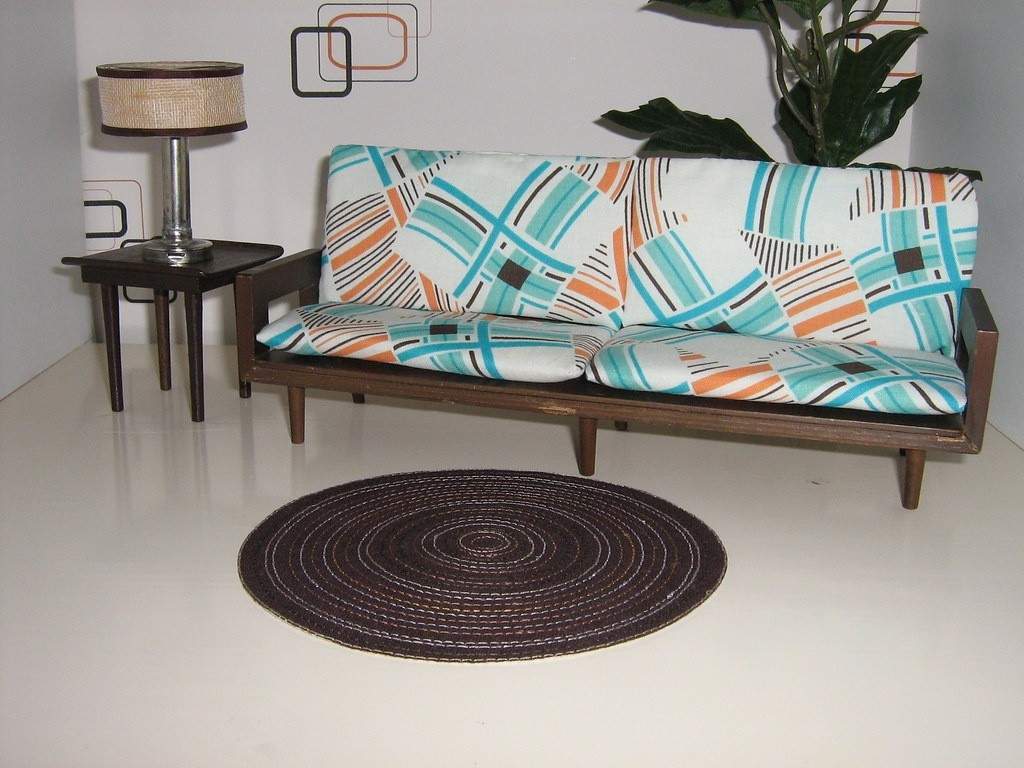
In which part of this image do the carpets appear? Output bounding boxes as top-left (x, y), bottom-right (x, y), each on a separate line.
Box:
top-left (237, 467), bottom-right (729, 662)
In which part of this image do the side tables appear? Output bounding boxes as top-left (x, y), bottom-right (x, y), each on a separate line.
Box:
top-left (61, 239), bottom-right (284, 422)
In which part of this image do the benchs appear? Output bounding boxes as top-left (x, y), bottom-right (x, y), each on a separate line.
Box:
top-left (235, 145), bottom-right (999, 510)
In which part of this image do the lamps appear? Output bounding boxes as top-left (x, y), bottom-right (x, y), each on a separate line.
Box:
top-left (96, 58), bottom-right (248, 263)
